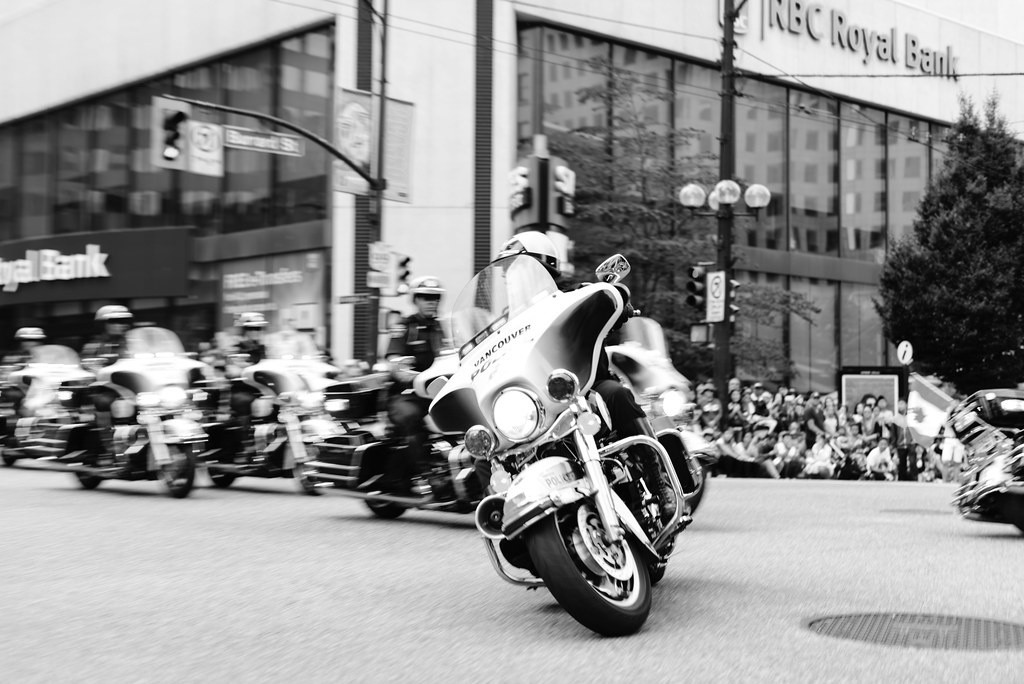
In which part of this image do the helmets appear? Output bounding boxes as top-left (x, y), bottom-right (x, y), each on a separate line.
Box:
top-left (14, 328), bottom-right (45, 339)
top-left (408, 279), bottom-right (443, 296)
top-left (94, 305), bottom-right (133, 321)
top-left (235, 313), bottom-right (265, 328)
top-left (496, 232), bottom-right (563, 275)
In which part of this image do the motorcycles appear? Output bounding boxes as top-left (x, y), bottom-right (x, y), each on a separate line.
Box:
top-left (425, 250), bottom-right (704, 637)
top-left (947, 389), bottom-right (1024, 529)
top-left (0, 327), bottom-right (478, 519)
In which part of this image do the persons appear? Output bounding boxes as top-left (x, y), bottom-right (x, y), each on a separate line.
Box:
top-left (694, 376), bottom-right (928, 482)
top-left (0, 326), bottom-right (58, 435)
top-left (932, 424), bottom-right (950, 468)
top-left (494, 230), bottom-right (680, 532)
top-left (198, 309), bottom-right (330, 466)
top-left (79, 304), bottom-right (182, 453)
top-left (385, 276), bottom-right (456, 497)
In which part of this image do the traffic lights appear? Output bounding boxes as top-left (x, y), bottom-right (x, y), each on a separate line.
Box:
top-left (686, 264), bottom-right (710, 308)
top-left (725, 272), bottom-right (741, 320)
top-left (393, 252), bottom-right (411, 293)
top-left (152, 97), bottom-right (193, 171)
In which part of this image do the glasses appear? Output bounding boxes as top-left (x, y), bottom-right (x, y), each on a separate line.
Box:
top-left (417, 294), bottom-right (440, 301)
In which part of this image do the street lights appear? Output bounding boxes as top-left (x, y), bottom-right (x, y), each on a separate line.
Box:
top-left (678, 0), bottom-right (774, 409)
